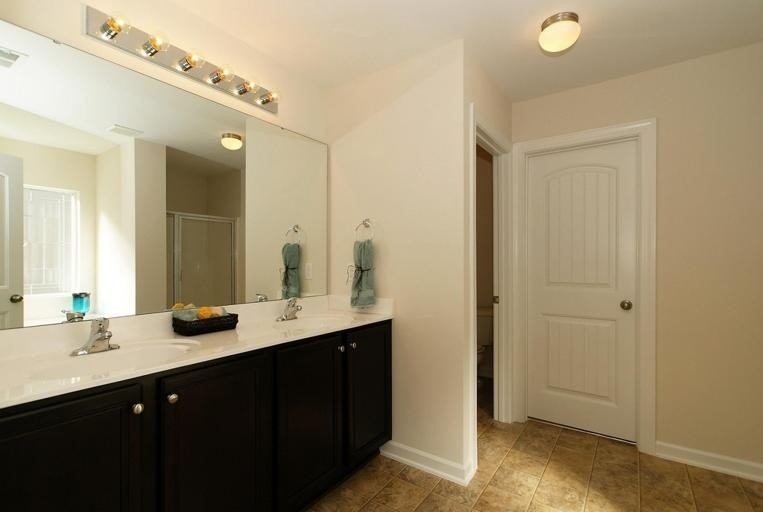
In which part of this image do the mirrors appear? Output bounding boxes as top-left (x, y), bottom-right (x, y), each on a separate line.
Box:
top-left (0, 18), bottom-right (334, 329)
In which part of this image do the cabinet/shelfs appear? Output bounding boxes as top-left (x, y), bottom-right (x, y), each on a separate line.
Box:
top-left (0, 347), bottom-right (279, 511)
top-left (277, 317), bottom-right (392, 512)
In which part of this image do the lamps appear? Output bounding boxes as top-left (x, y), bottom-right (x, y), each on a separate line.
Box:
top-left (222, 132), bottom-right (245, 150)
top-left (88, 6), bottom-right (282, 113)
top-left (536, 11), bottom-right (580, 55)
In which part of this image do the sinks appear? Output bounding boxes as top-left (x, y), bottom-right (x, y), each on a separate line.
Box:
top-left (254, 314), bottom-right (353, 342)
top-left (0, 338), bottom-right (199, 403)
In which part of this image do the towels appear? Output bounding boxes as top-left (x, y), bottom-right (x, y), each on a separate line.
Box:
top-left (277, 242), bottom-right (302, 299)
top-left (350, 242), bottom-right (375, 306)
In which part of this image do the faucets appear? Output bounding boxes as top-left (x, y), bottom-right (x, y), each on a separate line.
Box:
top-left (276, 296), bottom-right (306, 321)
top-left (72, 316), bottom-right (122, 356)
top-left (255, 293), bottom-right (268, 303)
top-left (58, 308), bottom-right (86, 324)
